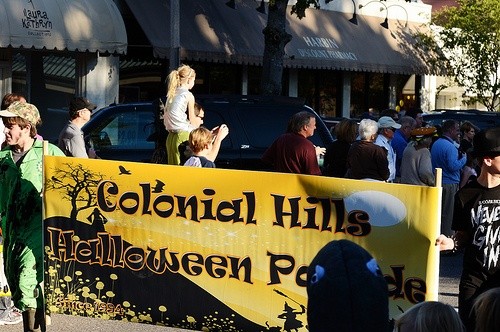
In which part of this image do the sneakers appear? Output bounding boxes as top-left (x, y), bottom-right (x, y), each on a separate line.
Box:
top-left (1, 308), bottom-right (23, 325)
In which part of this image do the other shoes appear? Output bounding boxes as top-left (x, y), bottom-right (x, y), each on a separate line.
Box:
top-left (440, 250), bottom-right (458, 256)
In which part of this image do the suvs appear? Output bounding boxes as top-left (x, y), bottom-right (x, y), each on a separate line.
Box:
top-left (421, 108), bottom-right (500, 145)
top-left (80, 96), bottom-right (334, 174)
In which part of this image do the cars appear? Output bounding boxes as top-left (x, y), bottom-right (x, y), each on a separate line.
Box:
top-left (323, 116), bottom-right (361, 140)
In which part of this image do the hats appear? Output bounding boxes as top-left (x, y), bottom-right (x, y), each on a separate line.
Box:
top-left (473, 127), bottom-right (500, 154)
top-left (0, 101), bottom-right (40, 125)
top-left (378, 116), bottom-right (402, 129)
top-left (410, 126), bottom-right (439, 141)
top-left (69, 96), bottom-right (97, 111)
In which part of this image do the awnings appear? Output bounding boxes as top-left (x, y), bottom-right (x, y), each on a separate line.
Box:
top-left (0, 0), bottom-right (127, 56)
top-left (118, 0), bottom-right (455, 77)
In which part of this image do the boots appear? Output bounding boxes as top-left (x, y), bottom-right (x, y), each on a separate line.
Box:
top-left (22, 310), bottom-right (42, 332)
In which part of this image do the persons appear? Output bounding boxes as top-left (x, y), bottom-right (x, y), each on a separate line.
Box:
top-left (436, 129), bottom-right (500, 324)
top-left (0, 101), bottom-right (66, 332)
top-left (0, 94), bottom-right (26, 324)
top-left (468, 288), bottom-right (500, 332)
top-left (164, 66), bottom-right (197, 133)
top-left (60, 97), bottom-right (97, 159)
top-left (178, 127), bottom-right (215, 167)
top-left (166, 104), bottom-right (228, 164)
top-left (267, 112), bottom-right (322, 175)
top-left (322, 105), bottom-right (475, 257)
top-left (395, 302), bottom-right (464, 332)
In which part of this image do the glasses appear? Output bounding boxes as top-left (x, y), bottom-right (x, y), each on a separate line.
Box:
top-left (471, 131), bottom-right (476, 133)
top-left (387, 127), bottom-right (396, 132)
top-left (197, 115), bottom-right (204, 120)
